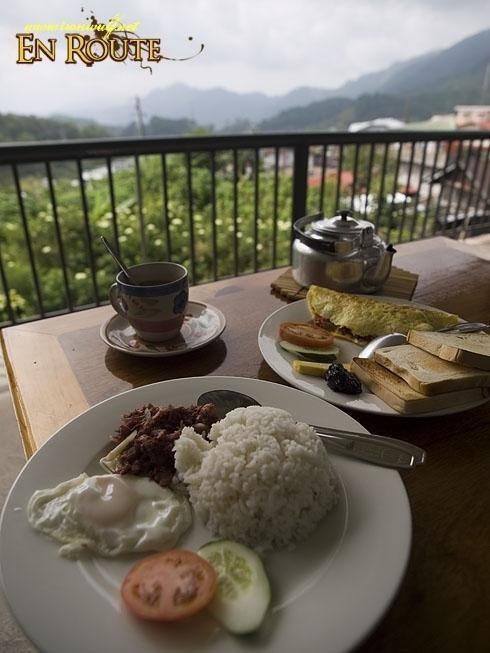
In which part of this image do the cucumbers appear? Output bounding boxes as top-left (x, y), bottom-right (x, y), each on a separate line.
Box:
top-left (190, 539), bottom-right (273, 636)
top-left (280, 340), bottom-right (340, 360)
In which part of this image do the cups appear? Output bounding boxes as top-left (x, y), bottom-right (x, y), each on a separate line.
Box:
top-left (107, 262), bottom-right (190, 340)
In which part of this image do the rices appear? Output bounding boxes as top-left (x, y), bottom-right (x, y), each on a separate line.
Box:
top-left (171, 404), bottom-right (340, 557)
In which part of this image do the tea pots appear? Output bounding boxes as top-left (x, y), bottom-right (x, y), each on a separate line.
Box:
top-left (289, 208), bottom-right (398, 294)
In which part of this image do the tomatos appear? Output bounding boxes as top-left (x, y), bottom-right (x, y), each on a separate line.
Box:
top-left (122, 548), bottom-right (218, 621)
top-left (279, 321), bottom-right (333, 348)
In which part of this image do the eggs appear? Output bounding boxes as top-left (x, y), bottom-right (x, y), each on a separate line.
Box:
top-left (29, 470), bottom-right (193, 560)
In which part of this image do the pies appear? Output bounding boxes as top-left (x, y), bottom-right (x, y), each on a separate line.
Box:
top-left (307, 284), bottom-right (459, 348)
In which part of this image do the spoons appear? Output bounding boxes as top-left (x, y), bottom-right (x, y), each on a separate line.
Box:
top-left (197, 387), bottom-right (428, 470)
top-left (356, 321), bottom-right (487, 361)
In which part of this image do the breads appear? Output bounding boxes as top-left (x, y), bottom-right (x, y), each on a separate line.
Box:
top-left (352, 329), bottom-right (490, 415)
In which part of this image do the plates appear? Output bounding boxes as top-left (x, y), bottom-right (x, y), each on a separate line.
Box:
top-left (2, 374), bottom-right (416, 650)
top-left (253, 292), bottom-right (490, 416)
top-left (97, 299), bottom-right (227, 360)
